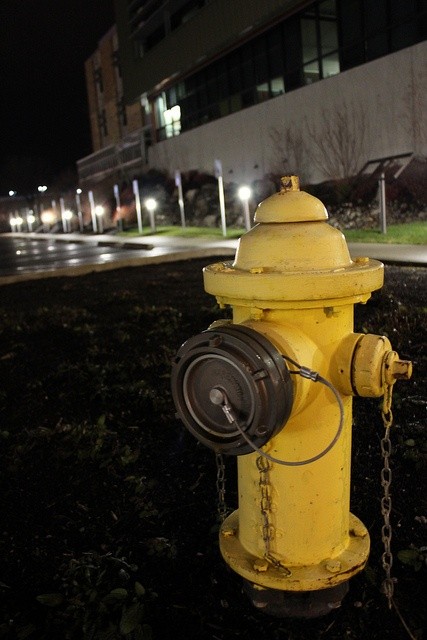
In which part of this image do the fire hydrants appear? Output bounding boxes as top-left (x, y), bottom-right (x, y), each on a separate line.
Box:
top-left (169, 173), bottom-right (414, 622)
